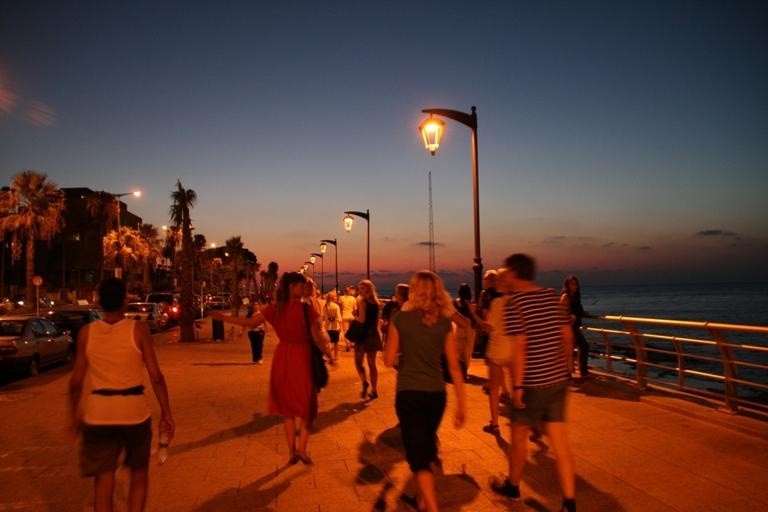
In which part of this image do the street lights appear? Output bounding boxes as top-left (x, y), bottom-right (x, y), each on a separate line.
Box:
top-left (111, 193), bottom-right (138, 250)
top-left (298, 239), bottom-right (338, 298)
top-left (419, 106), bottom-right (483, 302)
top-left (343, 210), bottom-right (370, 280)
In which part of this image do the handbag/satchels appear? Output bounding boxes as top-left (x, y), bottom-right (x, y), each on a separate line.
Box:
top-left (306, 335), bottom-right (329, 388)
top-left (345, 320), bottom-right (364, 345)
top-left (328, 313), bottom-right (335, 321)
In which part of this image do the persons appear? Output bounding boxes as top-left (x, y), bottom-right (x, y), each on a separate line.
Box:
top-left (207, 251), bottom-right (588, 510)
top-left (67, 277), bottom-right (175, 511)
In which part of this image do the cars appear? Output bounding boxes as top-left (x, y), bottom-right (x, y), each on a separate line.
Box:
top-left (122, 291), bottom-right (233, 331)
top-left (0, 307), bottom-right (104, 377)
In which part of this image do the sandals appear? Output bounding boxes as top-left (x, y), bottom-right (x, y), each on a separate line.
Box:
top-left (483, 423), bottom-right (500, 435)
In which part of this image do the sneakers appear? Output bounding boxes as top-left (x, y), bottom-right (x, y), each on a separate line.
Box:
top-left (288, 450), bottom-right (312, 464)
top-left (492, 480), bottom-right (520, 501)
top-left (361, 380), bottom-right (378, 399)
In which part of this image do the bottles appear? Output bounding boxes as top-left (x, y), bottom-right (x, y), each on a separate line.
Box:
top-left (158, 432), bottom-right (169, 466)
